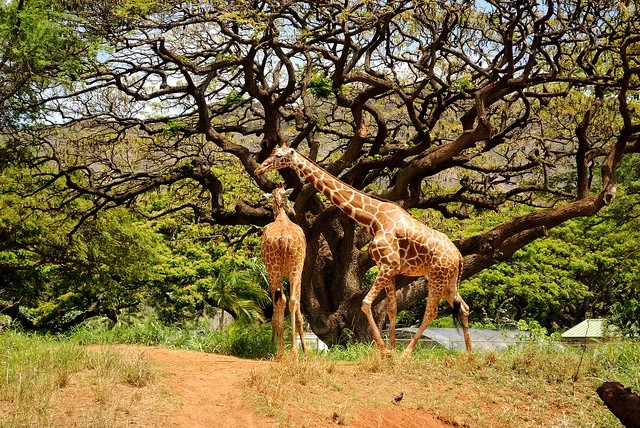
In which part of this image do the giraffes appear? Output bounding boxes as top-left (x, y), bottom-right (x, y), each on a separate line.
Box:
top-left (261, 182), bottom-right (307, 360)
top-left (253, 140), bottom-right (472, 361)
